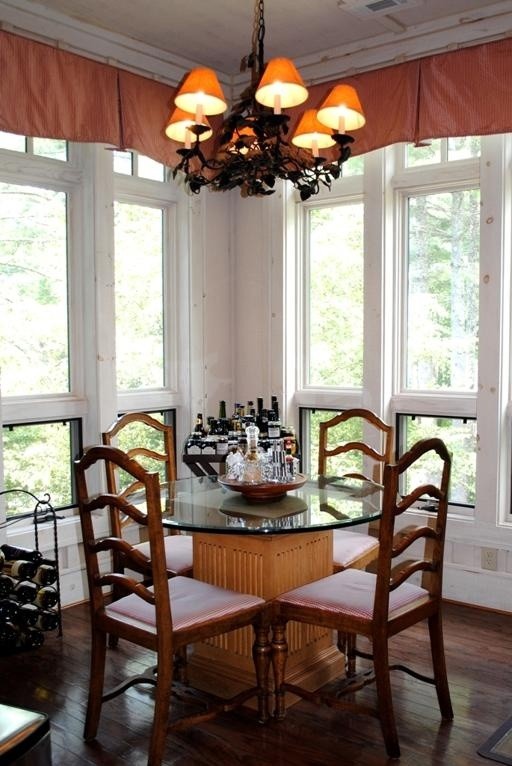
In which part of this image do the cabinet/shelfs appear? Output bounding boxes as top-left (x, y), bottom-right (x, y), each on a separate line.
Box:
top-left (0, 489), bottom-right (63, 652)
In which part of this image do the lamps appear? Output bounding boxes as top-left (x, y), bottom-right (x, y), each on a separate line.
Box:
top-left (164, 0), bottom-right (366, 202)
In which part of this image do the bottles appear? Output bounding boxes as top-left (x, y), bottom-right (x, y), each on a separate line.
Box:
top-left (185, 395), bottom-right (297, 457)
top-left (226, 423), bottom-right (295, 484)
top-left (0, 544), bottom-right (59, 655)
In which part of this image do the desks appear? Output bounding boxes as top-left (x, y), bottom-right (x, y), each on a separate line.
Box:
top-left (183, 454), bottom-right (300, 476)
top-left (124, 475), bottom-right (402, 718)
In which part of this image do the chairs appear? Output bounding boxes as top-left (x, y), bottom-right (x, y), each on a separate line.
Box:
top-left (102, 413), bottom-right (194, 662)
top-left (75, 446), bottom-right (268, 766)
top-left (318, 409), bottom-right (394, 654)
top-left (269, 438), bottom-right (454, 758)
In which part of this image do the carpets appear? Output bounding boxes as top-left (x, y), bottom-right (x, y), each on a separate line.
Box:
top-left (477, 716), bottom-right (512, 766)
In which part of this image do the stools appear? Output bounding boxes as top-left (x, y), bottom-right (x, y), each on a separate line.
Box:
top-left (0, 702), bottom-right (52, 766)
